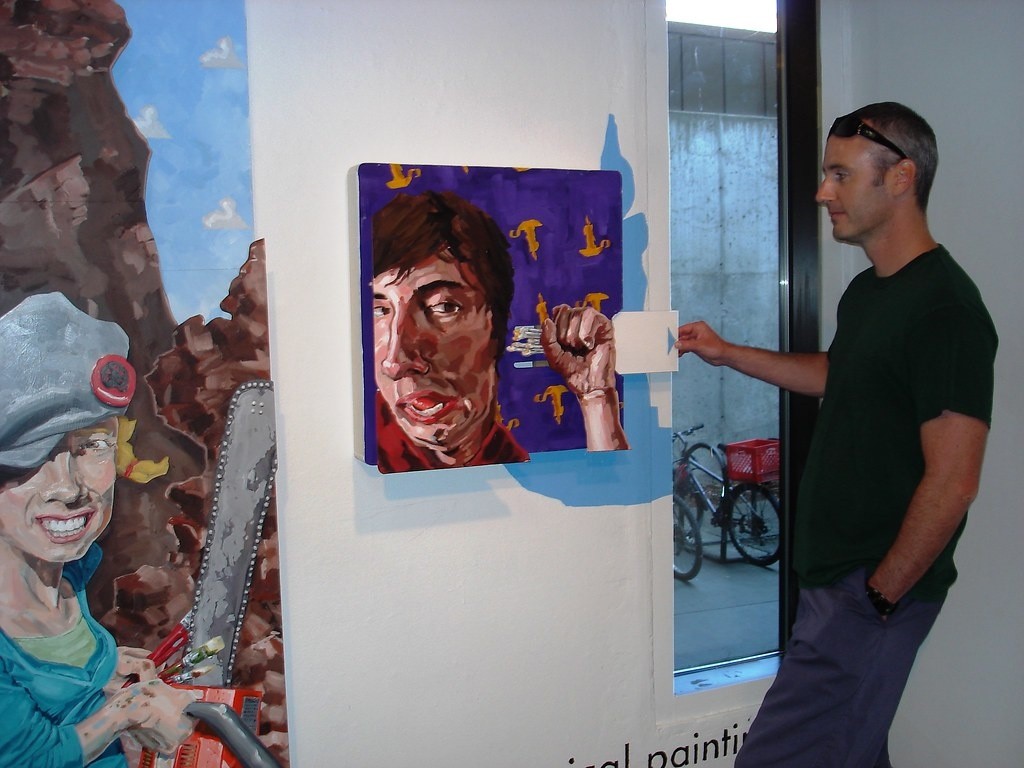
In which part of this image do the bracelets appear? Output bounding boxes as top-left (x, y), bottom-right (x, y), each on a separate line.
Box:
top-left (866, 584), bottom-right (897, 616)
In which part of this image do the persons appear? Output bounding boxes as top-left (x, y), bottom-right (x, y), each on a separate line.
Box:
top-left (675, 100), bottom-right (1000, 768)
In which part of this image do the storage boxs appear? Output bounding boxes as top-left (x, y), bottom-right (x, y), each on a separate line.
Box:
top-left (726, 439), bottom-right (779, 484)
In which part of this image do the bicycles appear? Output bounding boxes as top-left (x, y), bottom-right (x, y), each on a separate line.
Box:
top-left (672, 424), bottom-right (783, 581)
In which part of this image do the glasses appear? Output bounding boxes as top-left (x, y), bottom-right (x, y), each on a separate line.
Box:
top-left (827, 117), bottom-right (907, 160)
